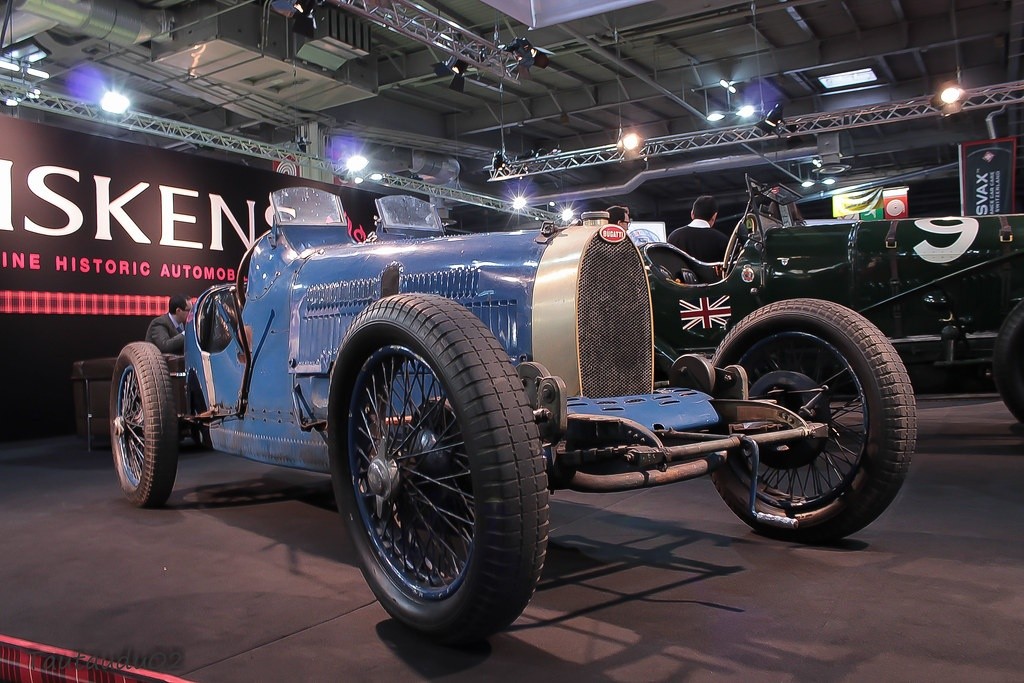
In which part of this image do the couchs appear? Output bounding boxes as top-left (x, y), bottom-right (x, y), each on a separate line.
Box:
top-left (71, 353), bottom-right (186, 453)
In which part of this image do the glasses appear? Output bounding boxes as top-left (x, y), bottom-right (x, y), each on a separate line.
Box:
top-left (622, 221), bottom-right (631, 227)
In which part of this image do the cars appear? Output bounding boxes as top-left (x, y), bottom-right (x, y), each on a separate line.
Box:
top-left (632, 211), bottom-right (1024, 429)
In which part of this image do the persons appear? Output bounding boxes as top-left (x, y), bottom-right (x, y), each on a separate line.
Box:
top-left (606, 205), bottom-right (630, 233)
top-left (667, 196), bottom-right (730, 264)
top-left (145, 295), bottom-right (193, 356)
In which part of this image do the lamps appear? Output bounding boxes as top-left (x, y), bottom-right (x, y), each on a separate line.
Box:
top-left (293, 0), bottom-right (316, 16)
top-left (765, 103), bottom-right (786, 127)
top-left (450, 59), bottom-right (469, 75)
top-left (801, 177), bottom-right (836, 188)
top-left (503, 37), bottom-right (555, 69)
